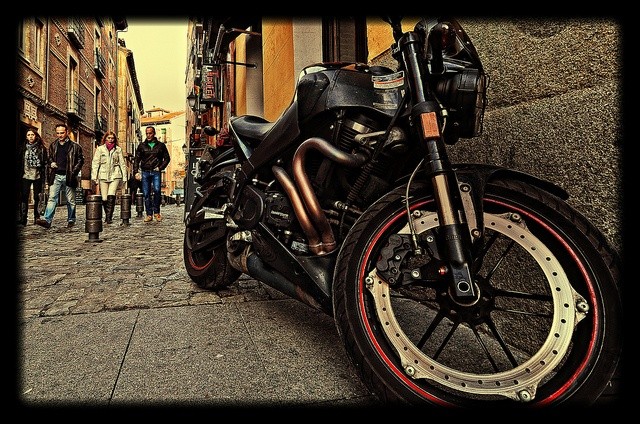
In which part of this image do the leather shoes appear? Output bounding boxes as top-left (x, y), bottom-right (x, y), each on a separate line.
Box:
top-left (36, 218), bottom-right (51, 228)
top-left (68, 220), bottom-right (75, 227)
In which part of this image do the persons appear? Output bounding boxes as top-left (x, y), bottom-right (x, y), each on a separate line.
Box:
top-left (133, 127), bottom-right (170, 222)
top-left (16, 128), bottom-right (48, 227)
top-left (37, 124), bottom-right (84, 229)
top-left (91, 131), bottom-right (128, 224)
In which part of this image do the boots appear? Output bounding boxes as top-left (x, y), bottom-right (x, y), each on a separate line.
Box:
top-left (102, 200), bottom-right (108, 223)
top-left (107, 195), bottom-right (117, 225)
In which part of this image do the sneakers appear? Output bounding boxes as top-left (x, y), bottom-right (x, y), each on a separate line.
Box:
top-left (154, 214), bottom-right (163, 222)
top-left (144, 216), bottom-right (154, 223)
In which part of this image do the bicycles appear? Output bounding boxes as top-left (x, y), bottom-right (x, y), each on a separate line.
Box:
top-left (183, 14), bottom-right (621, 411)
